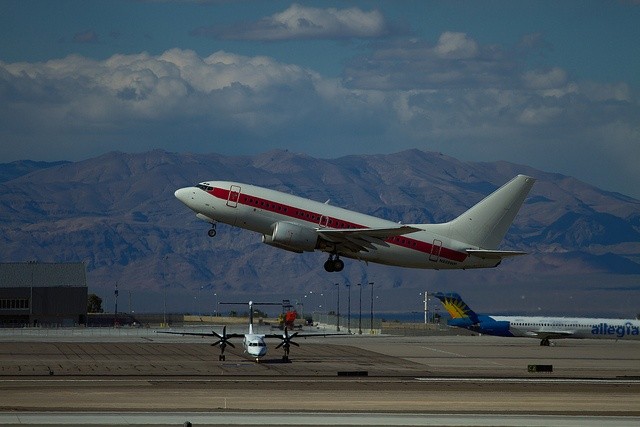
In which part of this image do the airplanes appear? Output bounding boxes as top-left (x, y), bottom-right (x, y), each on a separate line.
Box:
top-left (430, 292), bottom-right (640, 346)
top-left (210, 301), bottom-right (299, 363)
top-left (174, 173), bottom-right (536, 272)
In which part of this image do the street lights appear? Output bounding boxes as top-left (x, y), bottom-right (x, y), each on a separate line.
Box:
top-left (335, 284), bottom-right (340, 331)
top-left (369, 283), bottom-right (374, 335)
top-left (357, 284), bottom-right (362, 334)
top-left (346, 285), bottom-right (351, 332)
